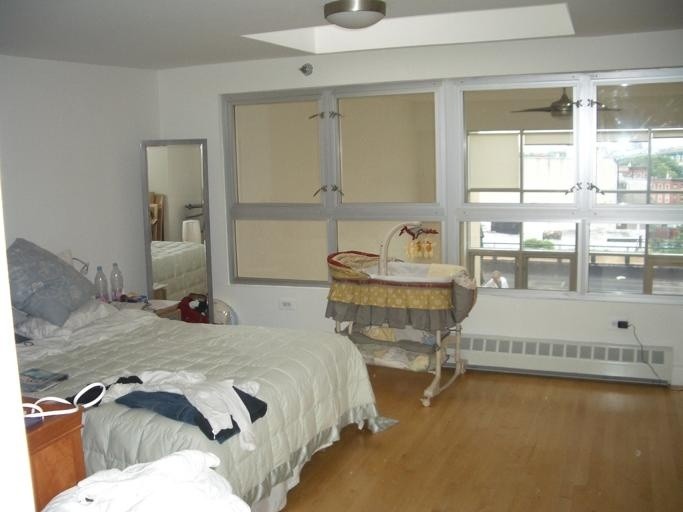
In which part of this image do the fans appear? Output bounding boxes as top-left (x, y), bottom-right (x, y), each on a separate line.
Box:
top-left (509, 86), bottom-right (622, 112)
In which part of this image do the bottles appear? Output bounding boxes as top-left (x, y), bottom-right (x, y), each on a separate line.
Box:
top-left (94, 266), bottom-right (108, 302)
top-left (110, 263), bottom-right (122, 300)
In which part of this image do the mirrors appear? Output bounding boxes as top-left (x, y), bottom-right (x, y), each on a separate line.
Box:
top-left (143, 138), bottom-right (216, 324)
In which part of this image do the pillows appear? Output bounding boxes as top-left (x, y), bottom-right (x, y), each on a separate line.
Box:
top-left (13, 298), bottom-right (118, 345)
top-left (6, 236), bottom-right (104, 326)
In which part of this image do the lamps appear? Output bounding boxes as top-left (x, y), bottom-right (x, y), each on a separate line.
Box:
top-left (323, 0), bottom-right (387, 29)
top-left (550, 110), bottom-right (574, 118)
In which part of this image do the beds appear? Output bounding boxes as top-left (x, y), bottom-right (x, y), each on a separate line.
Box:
top-left (151, 240), bottom-right (206, 298)
top-left (9, 238), bottom-right (402, 511)
top-left (326, 220), bottom-right (472, 407)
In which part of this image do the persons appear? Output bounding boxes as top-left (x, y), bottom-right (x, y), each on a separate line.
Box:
top-left (485, 271), bottom-right (509, 288)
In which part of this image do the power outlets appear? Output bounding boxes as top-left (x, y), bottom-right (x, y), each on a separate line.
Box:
top-left (608, 316), bottom-right (628, 332)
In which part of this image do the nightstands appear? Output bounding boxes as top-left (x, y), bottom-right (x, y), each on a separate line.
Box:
top-left (141, 299), bottom-right (181, 321)
top-left (19, 396), bottom-right (88, 511)
